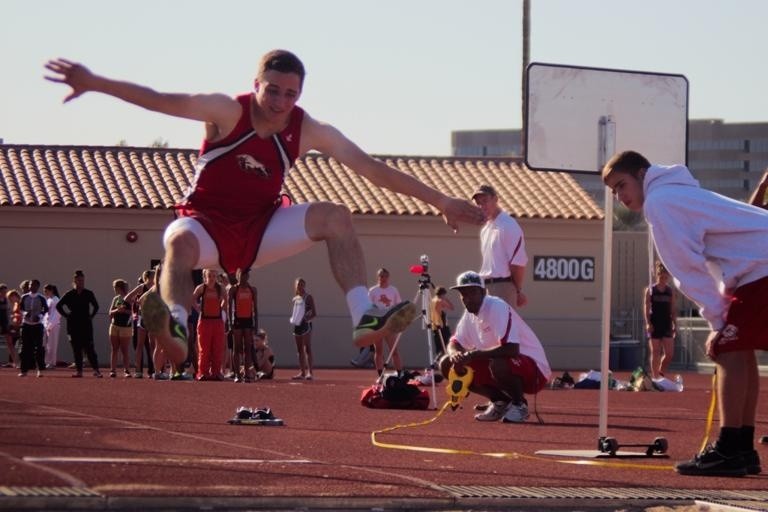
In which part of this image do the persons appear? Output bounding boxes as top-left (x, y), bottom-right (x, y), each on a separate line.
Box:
top-left (131, 277), bottom-right (156, 377)
top-left (473, 185), bottom-right (528, 311)
top-left (644, 262), bottom-right (679, 378)
top-left (139, 265), bottom-right (189, 379)
top-left (0, 283), bottom-right (17, 370)
top-left (20, 280), bottom-right (29, 293)
top-left (2, 290), bottom-right (24, 368)
top-left (439, 270), bottom-right (551, 423)
top-left (44, 284), bottom-right (63, 368)
top-left (123, 270), bottom-right (157, 377)
top-left (228, 269), bottom-right (259, 381)
top-left (193, 269), bottom-right (228, 379)
top-left (255, 329), bottom-right (277, 379)
top-left (44, 50), bottom-right (487, 364)
top-left (291, 278), bottom-right (317, 379)
top-left (430, 287), bottom-right (455, 355)
top-left (108, 278), bottom-right (134, 376)
top-left (602, 151), bottom-right (768, 478)
top-left (18, 279), bottom-right (48, 377)
top-left (215, 273), bottom-right (239, 378)
top-left (56, 271), bottom-right (100, 377)
top-left (369, 269), bottom-right (403, 376)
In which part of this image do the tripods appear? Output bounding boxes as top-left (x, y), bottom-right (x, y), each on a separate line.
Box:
top-left (374, 254), bottom-right (463, 410)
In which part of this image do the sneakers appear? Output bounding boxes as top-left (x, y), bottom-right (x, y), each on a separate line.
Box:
top-left (180, 372), bottom-right (193, 380)
top-left (674, 444), bottom-right (748, 476)
top-left (37, 371), bottom-right (44, 377)
top-left (135, 369), bottom-right (143, 378)
top-left (16, 367), bottom-right (21, 371)
top-left (252, 408), bottom-right (271, 419)
top-left (212, 373), bottom-right (224, 381)
top-left (169, 372), bottom-right (180, 381)
top-left (110, 369), bottom-right (116, 377)
top-left (229, 372), bottom-right (235, 378)
top-left (503, 400), bottom-right (529, 424)
top-left (746, 450), bottom-right (761, 474)
top-left (124, 370), bottom-right (132, 378)
top-left (353, 301), bottom-right (416, 347)
top-left (46, 364), bottom-right (56, 369)
top-left (292, 375), bottom-right (305, 380)
top-left (235, 375), bottom-right (240, 382)
top-left (377, 377), bottom-right (383, 384)
top-left (198, 374), bottom-right (207, 381)
top-left (471, 183), bottom-right (496, 199)
top-left (306, 376), bottom-right (314, 381)
top-left (474, 401), bottom-right (509, 422)
top-left (552, 373), bottom-right (575, 389)
top-left (70, 363), bottom-right (78, 368)
top-left (142, 292), bottom-right (188, 365)
top-left (245, 376), bottom-right (257, 382)
top-left (93, 369), bottom-right (103, 378)
top-left (72, 372), bottom-right (83, 378)
top-left (237, 407), bottom-right (253, 420)
top-left (18, 372), bottom-right (28, 377)
top-left (2, 363), bottom-right (15, 368)
top-left (148, 371), bottom-right (168, 380)
top-left (575, 371), bottom-right (601, 389)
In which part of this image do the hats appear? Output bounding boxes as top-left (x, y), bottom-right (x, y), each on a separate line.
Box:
top-left (449, 271), bottom-right (485, 290)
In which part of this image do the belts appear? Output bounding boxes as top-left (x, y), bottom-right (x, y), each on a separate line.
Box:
top-left (483, 277), bottom-right (513, 284)
